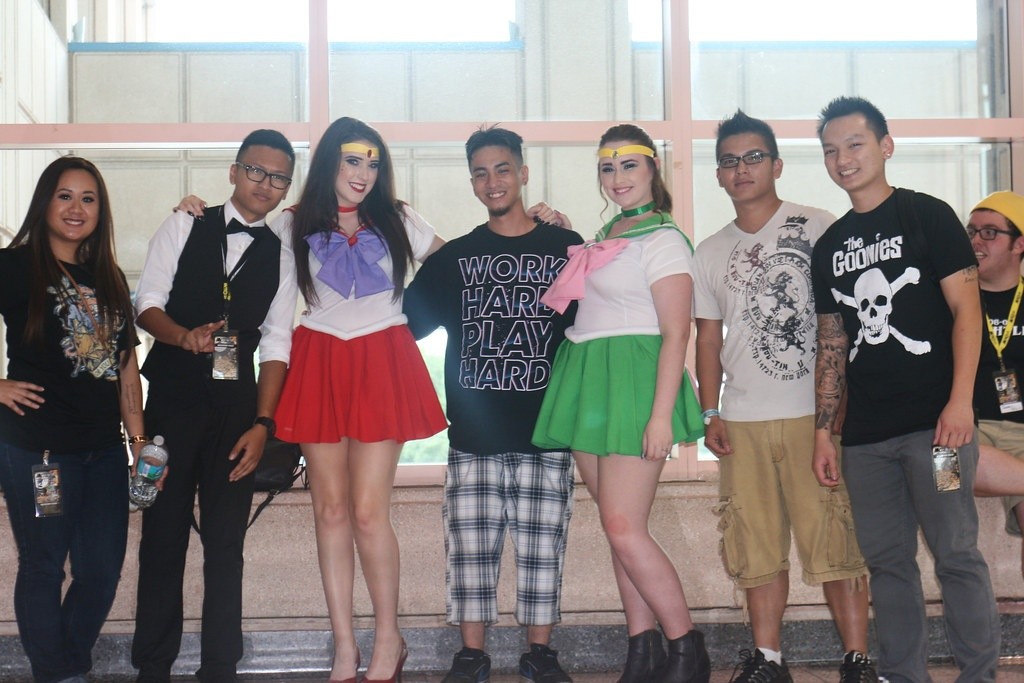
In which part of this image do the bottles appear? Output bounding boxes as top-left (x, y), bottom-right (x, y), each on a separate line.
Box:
top-left (129, 436), bottom-right (169, 506)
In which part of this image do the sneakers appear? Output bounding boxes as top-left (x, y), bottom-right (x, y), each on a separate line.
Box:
top-left (520, 644), bottom-right (573, 683)
top-left (440, 647), bottom-right (491, 683)
top-left (838, 650), bottom-right (878, 683)
top-left (729, 648), bottom-right (793, 683)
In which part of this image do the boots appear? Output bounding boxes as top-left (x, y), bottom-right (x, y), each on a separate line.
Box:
top-left (617, 629), bottom-right (667, 683)
top-left (651, 630), bottom-right (710, 683)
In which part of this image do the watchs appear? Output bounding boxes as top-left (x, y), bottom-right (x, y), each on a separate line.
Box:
top-left (254, 417), bottom-right (275, 440)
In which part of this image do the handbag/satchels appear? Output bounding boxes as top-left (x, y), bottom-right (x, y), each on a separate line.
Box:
top-left (253, 442), bottom-right (303, 492)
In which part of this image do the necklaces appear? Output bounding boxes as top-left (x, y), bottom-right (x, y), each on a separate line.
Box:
top-left (338, 207), bottom-right (358, 212)
top-left (55, 261), bottom-right (120, 381)
top-left (621, 201), bottom-right (655, 217)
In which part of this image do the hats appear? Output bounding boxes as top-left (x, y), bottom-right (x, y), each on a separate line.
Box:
top-left (969, 191), bottom-right (1024, 237)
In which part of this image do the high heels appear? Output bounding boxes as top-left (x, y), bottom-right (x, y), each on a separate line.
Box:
top-left (327, 646), bottom-right (361, 683)
top-left (361, 637), bottom-right (408, 683)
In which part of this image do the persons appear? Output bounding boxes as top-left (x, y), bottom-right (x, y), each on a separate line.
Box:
top-left (129, 128), bottom-right (295, 683)
top-left (0, 158), bottom-right (168, 683)
top-left (403, 128), bottom-right (585, 683)
top-left (559, 123), bottom-right (711, 682)
top-left (172, 117), bottom-right (559, 683)
top-left (692, 109), bottom-right (882, 682)
top-left (965, 191), bottom-right (1024, 578)
top-left (812, 96), bottom-right (1000, 682)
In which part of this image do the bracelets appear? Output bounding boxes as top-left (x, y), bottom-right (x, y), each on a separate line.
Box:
top-left (129, 435), bottom-right (146, 446)
top-left (702, 410), bottom-right (719, 418)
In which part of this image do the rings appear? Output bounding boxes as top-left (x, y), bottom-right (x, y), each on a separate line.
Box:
top-left (662, 449), bottom-right (668, 451)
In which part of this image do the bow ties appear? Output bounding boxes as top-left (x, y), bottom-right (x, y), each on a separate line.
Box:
top-left (225, 217), bottom-right (266, 239)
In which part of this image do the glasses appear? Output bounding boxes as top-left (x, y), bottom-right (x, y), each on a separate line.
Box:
top-left (717, 151), bottom-right (771, 169)
top-left (966, 228), bottom-right (1021, 240)
top-left (236, 162), bottom-right (292, 190)
